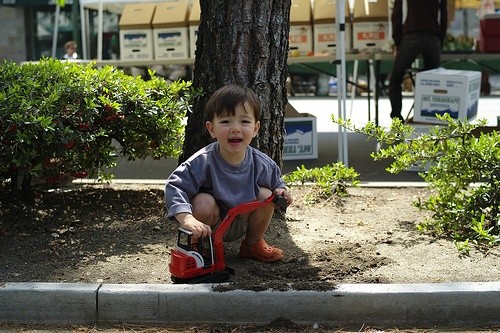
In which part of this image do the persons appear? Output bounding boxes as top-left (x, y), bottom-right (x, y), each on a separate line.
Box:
top-left (61, 41), bottom-right (81, 60)
top-left (388, 0), bottom-right (449, 122)
top-left (164, 84), bottom-right (293, 264)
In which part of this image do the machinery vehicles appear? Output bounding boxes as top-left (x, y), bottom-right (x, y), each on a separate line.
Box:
top-left (169, 192), bottom-right (289, 283)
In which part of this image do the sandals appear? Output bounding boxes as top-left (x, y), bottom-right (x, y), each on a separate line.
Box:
top-left (240, 237), bottom-right (283, 263)
